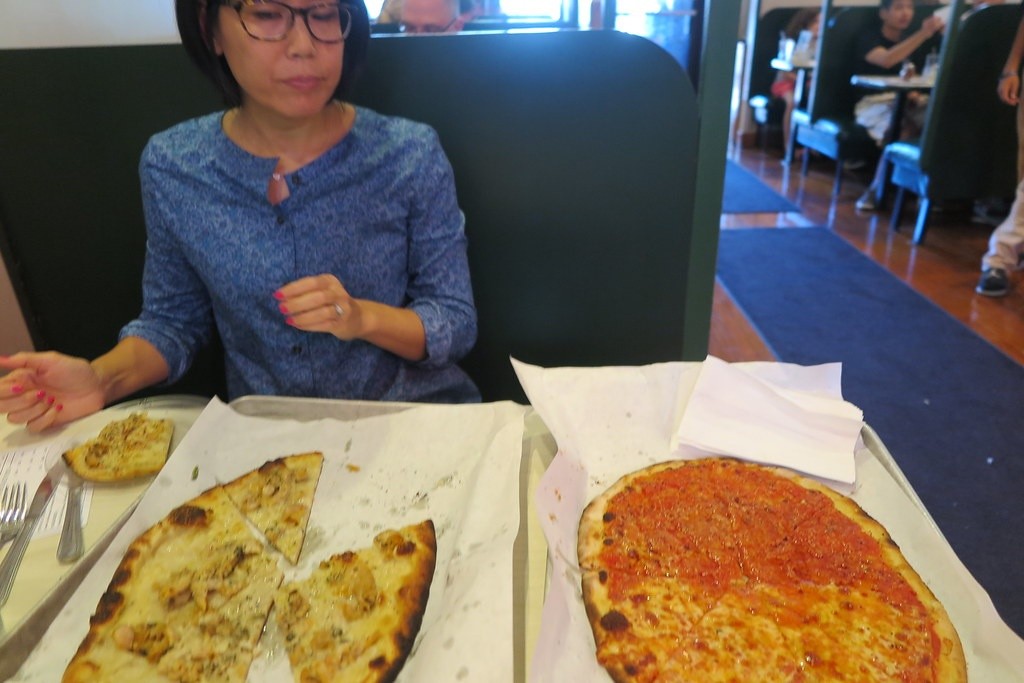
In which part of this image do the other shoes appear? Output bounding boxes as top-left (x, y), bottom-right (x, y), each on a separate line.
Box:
top-left (976, 266), bottom-right (1009, 296)
top-left (856, 189), bottom-right (876, 210)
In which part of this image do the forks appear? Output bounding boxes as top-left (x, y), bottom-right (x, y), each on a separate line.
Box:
top-left (0, 482), bottom-right (28, 553)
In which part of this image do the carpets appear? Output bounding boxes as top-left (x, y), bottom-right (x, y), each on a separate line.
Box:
top-left (717, 225), bottom-right (1023, 639)
top-left (721, 160), bottom-right (800, 212)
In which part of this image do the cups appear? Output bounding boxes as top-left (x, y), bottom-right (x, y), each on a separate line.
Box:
top-left (778, 37), bottom-right (794, 62)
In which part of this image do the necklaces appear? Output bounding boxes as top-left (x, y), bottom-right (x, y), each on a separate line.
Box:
top-left (238, 117), bottom-right (319, 180)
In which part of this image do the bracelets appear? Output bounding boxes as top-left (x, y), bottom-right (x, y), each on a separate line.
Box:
top-left (1000, 71), bottom-right (1017, 80)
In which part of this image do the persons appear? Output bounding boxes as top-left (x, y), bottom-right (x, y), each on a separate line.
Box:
top-left (845, 0), bottom-right (946, 210)
top-left (773, 6), bottom-right (825, 165)
top-left (0, 0), bottom-right (483, 434)
top-left (376, 0), bottom-right (466, 34)
top-left (977, 10), bottom-right (1024, 296)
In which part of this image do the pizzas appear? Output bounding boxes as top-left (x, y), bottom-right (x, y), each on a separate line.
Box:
top-left (62, 414), bottom-right (174, 480)
top-left (59, 452), bottom-right (437, 683)
top-left (575, 457), bottom-right (969, 683)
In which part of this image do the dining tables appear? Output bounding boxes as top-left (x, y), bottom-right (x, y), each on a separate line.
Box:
top-left (771, 59), bottom-right (814, 166)
top-left (0, 396), bottom-right (1024, 683)
top-left (850, 74), bottom-right (935, 229)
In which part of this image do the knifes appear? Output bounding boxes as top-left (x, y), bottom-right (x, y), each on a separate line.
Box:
top-left (0, 456), bottom-right (69, 608)
top-left (56, 468), bottom-right (84, 564)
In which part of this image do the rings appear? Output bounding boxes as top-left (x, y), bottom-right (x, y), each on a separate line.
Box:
top-left (334, 303), bottom-right (343, 319)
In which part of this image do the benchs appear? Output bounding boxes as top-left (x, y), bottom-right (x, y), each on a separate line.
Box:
top-left (0, 30), bottom-right (699, 403)
top-left (753, 3), bottom-right (1020, 243)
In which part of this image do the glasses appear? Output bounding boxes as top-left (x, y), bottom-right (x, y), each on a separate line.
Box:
top-left (215, 0), bottom-right (353, 43)
